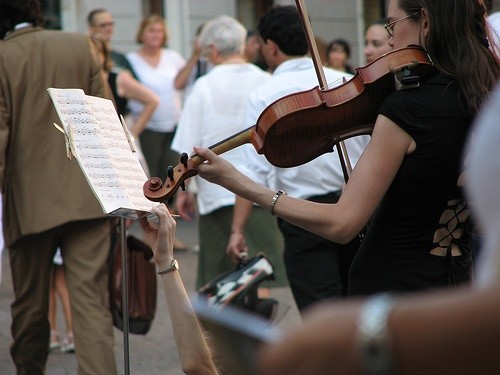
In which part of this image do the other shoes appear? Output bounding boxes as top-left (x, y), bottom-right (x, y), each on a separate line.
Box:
top-left (61, 335), bottom-right (75, 353)
top-left (50, 330), bottom-right (60, 349)
top-left (173, 239), bottom-right (187, 251)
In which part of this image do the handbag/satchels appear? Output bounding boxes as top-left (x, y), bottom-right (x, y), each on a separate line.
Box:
top-left (108, 229), bottom-right (157, 336)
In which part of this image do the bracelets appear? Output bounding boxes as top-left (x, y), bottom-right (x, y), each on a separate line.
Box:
top-left (268, 189), bottom-right (288, 216)
top-left (354, 295), bottom-right (391, 375)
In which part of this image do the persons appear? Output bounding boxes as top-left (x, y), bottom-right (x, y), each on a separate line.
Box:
top-left (1, 0), bottom-right (119, 374)
top-left (170, 15), bottom-right (290, 291)
top-left (126, 14), bottom-right (185, 221)
top-left (47, 248), bottom-right (74, 353)
top-left (193, 0), bottom-right (500, 298)
top-left (87, 8), bottom-right (160, 138)
top-left (226, 6), bottom-right (371, 311)
top-left (174, 23), bottom-right (391, 98)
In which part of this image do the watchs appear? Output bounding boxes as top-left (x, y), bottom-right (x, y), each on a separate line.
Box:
top-left (157, 260), bottom-right (179, 274)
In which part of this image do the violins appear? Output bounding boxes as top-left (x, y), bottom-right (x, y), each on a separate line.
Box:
top-left (144, 44), bottom-right (432, 203)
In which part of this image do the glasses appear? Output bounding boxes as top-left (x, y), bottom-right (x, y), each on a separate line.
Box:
top-left (385, 10), bottom-right (421, 38)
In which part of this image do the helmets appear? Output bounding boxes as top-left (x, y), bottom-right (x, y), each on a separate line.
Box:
top-left (199, 252), bottom-right (290, 326)
